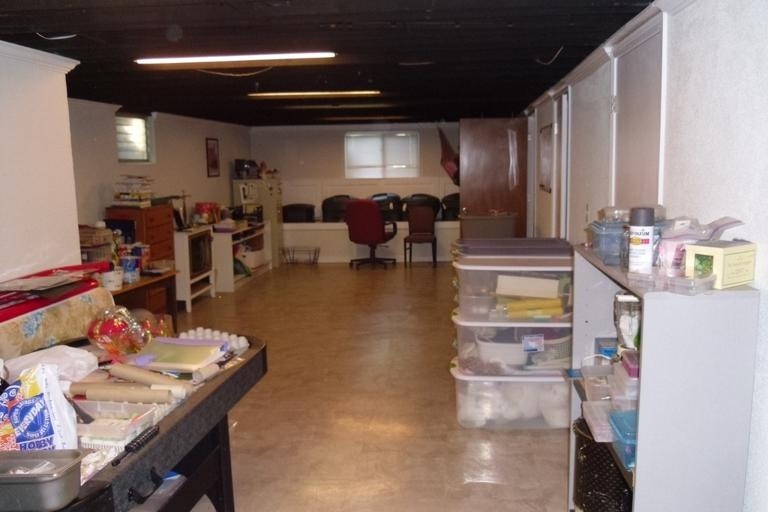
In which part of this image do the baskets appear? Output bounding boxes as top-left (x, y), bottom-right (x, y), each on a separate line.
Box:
top-left (573, 417), bottom-right (632, 512)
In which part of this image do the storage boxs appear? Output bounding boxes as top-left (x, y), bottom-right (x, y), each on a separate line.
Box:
top-left (449, 255), bottom-right (574, 325)
top-left (82, 246), bottom-right (112, 266)
top-left (575, 349), bottom-right (638, 472)
top-left (79, 227), bottom-right (113, 248)
top-left (0, 445), bottom-right (84, 511)
top-left (455, 208), bottom-right (572, 256)
top-left (587, 221), bottom-right (625, 262)
top-left (452, 308), bottom-right (572, 375)
top-left (450, 358), bottom-right (568, 431)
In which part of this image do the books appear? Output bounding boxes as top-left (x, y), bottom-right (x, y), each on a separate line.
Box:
top-left (134, 338), bottom-right (227, 372)
top-left (110, 172), bottom-right (150, 210)
top-left (494, 272), bottom-right (559, 299)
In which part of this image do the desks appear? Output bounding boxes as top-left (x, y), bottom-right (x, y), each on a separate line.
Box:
top-left (46, 333), bottom-right (268, 512)
top-left (111, 269), bottom-right (178, 334)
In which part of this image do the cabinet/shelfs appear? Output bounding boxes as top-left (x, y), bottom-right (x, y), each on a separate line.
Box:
top-left (106, 198), bottom-right (175, 312)
top-left (174, 225), bottom-right (216, 313)
top-left (566, 250), bottom-right (761, 511)
top-left (213, 221), bottom-right (271, 292)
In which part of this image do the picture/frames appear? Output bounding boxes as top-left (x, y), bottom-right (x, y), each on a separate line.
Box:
top-left (204, 138), bottom-right (221, 178)
top-left (537, 124), bottom-right (556, 199)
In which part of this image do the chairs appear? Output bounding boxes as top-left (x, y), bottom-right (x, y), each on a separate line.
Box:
top-left (343, 199), bottom-right (397, 270)
top-left (404, 205), bottom-right (437, 269)
